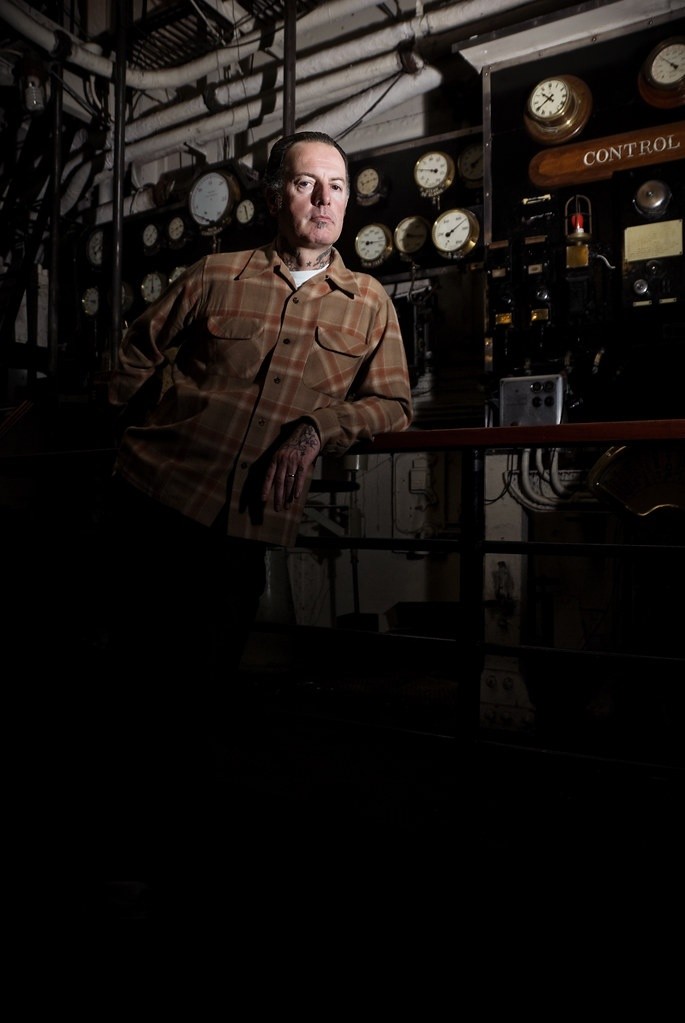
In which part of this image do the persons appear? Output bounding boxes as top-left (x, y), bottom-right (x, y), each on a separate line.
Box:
top-left (106, 132), bottom-right (410, 652)
